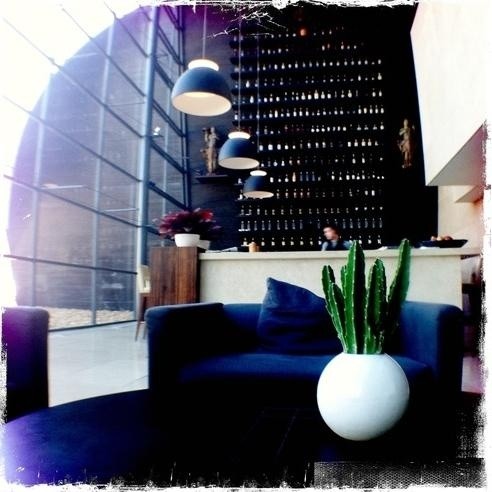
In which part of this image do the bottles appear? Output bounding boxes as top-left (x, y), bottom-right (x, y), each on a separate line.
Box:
top-left (230, 28), bottom-right (385, 251)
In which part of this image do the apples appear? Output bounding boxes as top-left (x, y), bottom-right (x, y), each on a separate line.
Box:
top-left (431, 235), bottom-right (453, 241)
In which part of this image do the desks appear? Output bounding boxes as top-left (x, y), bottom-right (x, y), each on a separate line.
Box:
top-left (1, 377), bottom-right (488, 486)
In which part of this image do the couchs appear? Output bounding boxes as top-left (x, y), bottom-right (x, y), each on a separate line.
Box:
top-left (144, 300), bottom-right (462, 448)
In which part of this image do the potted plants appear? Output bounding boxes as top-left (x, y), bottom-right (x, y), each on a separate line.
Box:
top-left (316, 238), bottom-right (413, 442)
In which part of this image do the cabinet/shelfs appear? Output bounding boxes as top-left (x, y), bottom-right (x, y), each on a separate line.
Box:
top-left (451, 186), bottom-right (483, 204)
top-left (408, 3), bottom-right (486, 186)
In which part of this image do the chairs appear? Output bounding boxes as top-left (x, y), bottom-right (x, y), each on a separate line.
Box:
top-left (2, 307), bottom-right (50, 421)
top-left (134, 265), bottom-right (150, 342)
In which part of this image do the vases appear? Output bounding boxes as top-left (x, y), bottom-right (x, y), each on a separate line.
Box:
top-left (174, 234), bottom-right (200, 248)
top-left (200, 225), bottom-right (224, 241)
top-left (198, 240), bottom-right (209, 249)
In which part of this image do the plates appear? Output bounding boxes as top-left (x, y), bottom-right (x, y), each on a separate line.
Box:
top-left (417, 239), bottom-right (467, 248)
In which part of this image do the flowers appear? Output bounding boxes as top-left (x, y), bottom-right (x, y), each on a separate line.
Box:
top-left (157, 206), bottom-right (213, 239)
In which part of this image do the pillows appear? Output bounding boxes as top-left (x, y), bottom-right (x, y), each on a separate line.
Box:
top-left (255, 278), bottom-right (340, 351)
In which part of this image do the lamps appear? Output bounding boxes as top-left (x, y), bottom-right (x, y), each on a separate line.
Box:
top-left (217, 6), bottom-right (260, 169)
top-left (242, 45), bottom-right (275, 200)
top-left (171, 0), bottom-right (232, 117)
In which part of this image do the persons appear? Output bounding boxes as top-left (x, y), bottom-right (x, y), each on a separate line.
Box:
top-left (204, 126), bottom-right (220, 175)
top-left (320, 225), bottom-right (352, 251)
top-left (399, 119), bottom-right (415, 168)
